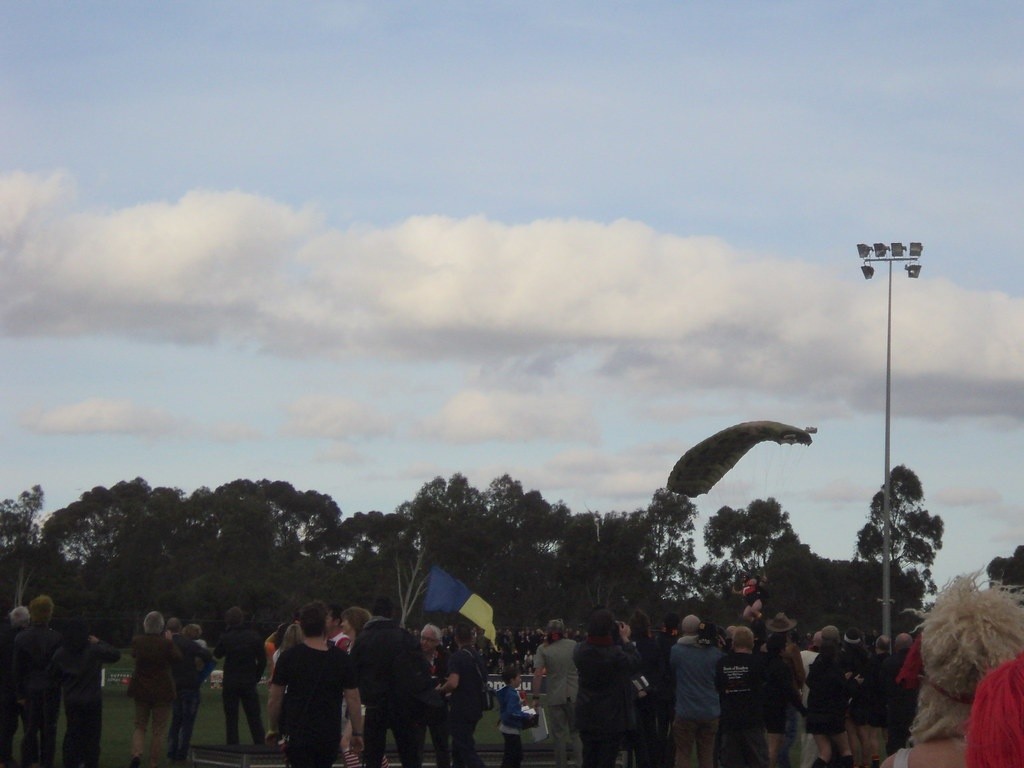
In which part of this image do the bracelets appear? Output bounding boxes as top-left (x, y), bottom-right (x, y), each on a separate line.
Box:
top-left (352, 732), bottom-right (362, 736)
top-left (533, 696), bottom-right (539, 699)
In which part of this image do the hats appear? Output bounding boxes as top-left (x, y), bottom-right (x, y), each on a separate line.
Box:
top-left (766, 612), bottom-right (798, 632)
top-left (628, 607), bottom-right (652, 632)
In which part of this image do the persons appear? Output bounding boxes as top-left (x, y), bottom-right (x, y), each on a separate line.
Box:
top-left (533, 620), bottom-right (583, 768)
top-left (264, 606), bottom-right (424, 768)
top-left (407, 626), bottom-right (587, 676)
top-left (413, 624), bottom-right (489, 768)
top-left (496, 668), bottom-right (535, 768)
top-left (46, 620), bottom-right (121, 768)
top-left (130, 611), bottom-right (217, 768)
top-left (878, 571), bottom-right (1024, 768)
top-left (573, 612), bottom-right (921, 768)
top-left (0, 594), bottom-right (64, 768)
top-left (213, 607), bottom-right (267, 745)
top-left (743, 572), bottom-right (770, 626)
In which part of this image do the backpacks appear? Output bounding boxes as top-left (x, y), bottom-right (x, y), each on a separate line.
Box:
top-left (845, 652), bottom-right (882, 699)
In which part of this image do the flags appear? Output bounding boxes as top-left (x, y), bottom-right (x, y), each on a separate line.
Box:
top-left (422, 565), bottom-right (499, 653)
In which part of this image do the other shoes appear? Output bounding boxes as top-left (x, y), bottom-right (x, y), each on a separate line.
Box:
top-left (165, 758), bottom-right (192, 768)
top-left (128, 755), bottom-right (141, 768)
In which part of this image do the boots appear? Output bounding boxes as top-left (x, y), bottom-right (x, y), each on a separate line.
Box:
top-left (839, 754), bottom-right (854, 768)
top-left (812, 757), bottom-right (828, 768)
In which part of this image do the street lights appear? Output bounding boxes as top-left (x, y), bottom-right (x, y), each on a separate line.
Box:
top-left (857, 243), bottom-right (922, 655)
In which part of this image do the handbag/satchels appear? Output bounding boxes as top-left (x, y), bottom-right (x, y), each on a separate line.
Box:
top-left (481, 684), bottom-right (494, 711)
top-left (644, 659), bottom-right (676, 695)
top-left (279, 734), bottom-right (295, 765)
